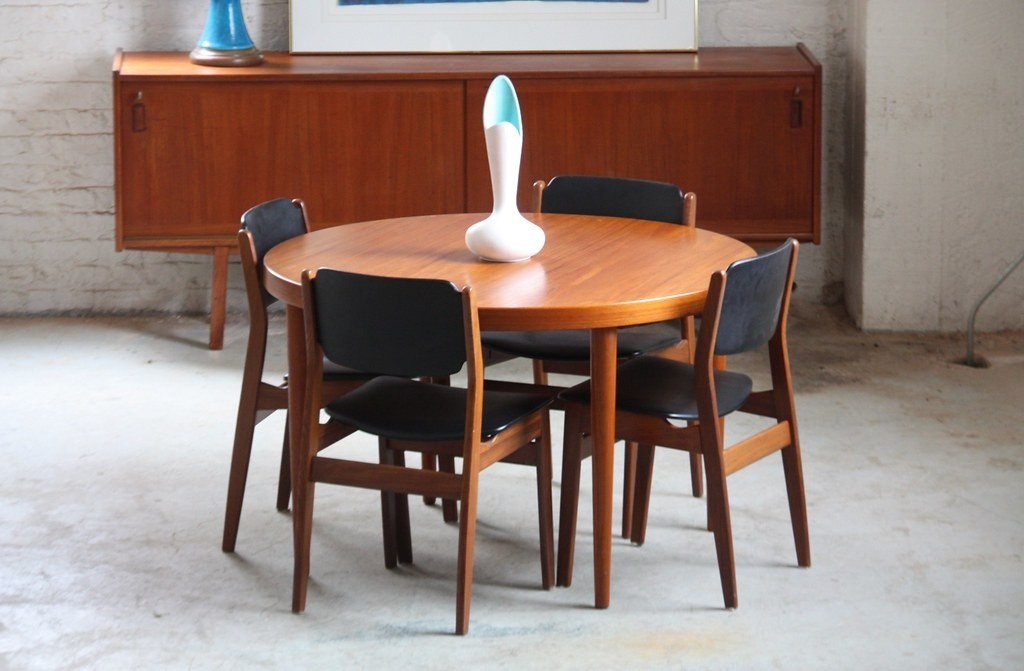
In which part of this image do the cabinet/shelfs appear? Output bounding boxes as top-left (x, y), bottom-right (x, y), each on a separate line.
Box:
top-left (111, 41), bottom-right (825, 351)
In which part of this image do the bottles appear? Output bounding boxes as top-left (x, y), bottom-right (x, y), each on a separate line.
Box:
top-left (464, 74), bottom-right (546, 263)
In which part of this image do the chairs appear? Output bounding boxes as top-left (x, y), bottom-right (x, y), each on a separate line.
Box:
top-left (285, 267), bottom-right (556, 634)
top-left (220, 196), bottom-right (457, 565)
top-left (423, 175), bottom-right (705, 539)
top-left (554, 236), bottom-right (813, 609)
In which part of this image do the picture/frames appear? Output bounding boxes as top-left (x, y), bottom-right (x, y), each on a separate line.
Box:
top-left (288, 0), bottom-right (700, 54)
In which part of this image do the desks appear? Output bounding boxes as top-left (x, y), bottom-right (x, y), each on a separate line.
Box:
top-left (263, 213), bottom-right (758, 610)
